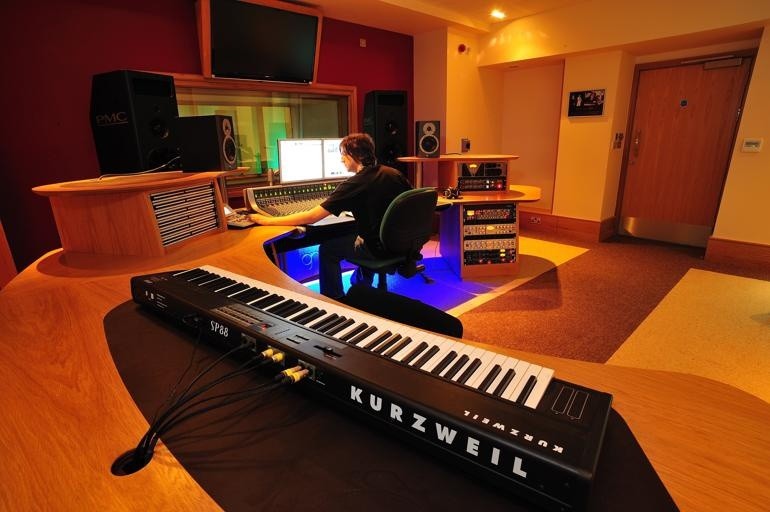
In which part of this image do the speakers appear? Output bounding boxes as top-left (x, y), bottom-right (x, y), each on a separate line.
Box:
top-left (414, 118), bottom-right (441, 158)
top-left (365, 88), bottom-right (409, 179)
top-left (174, 115), bottom-right (238, 172)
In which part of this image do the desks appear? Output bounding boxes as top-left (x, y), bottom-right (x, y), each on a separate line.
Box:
top-left (0, 154), bottom-right (770, 511)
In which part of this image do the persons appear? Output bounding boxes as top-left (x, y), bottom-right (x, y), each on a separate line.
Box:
top-left (584, 92), bottom-right (599, 109)
top-left (244, 131), bottom-right (416, 300)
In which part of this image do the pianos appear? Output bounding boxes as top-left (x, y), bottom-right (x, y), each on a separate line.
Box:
top-left (131, 265), bottom-right (613, 511)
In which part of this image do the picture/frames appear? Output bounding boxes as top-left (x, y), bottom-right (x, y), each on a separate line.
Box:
top-left (567, 88), bottom-right (606, 117)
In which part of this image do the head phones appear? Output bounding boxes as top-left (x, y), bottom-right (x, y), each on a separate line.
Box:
top-left (444, 187), bottom-right (461, 200)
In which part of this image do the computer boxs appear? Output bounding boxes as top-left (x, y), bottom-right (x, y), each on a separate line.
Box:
top-left (88, 66), bottom-right (179, 176)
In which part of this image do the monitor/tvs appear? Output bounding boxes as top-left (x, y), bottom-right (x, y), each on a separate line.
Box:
top-left (198, 0), bottom-right (325, 88)
top-left (276, 139), bottom-right (323, 184)
top-left (323, 138), bottom-right (357, 180)
